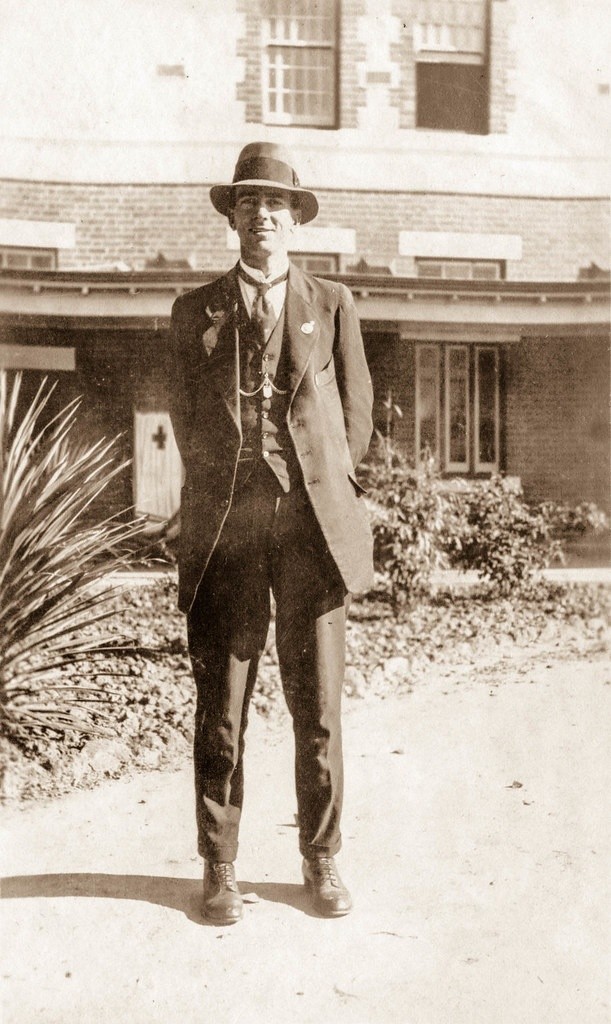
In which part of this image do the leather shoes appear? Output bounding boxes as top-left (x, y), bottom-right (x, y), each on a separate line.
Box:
top-left (302, 858), bottom-right (352, 914)
top-left (203, 862), bottom-right (243, 922)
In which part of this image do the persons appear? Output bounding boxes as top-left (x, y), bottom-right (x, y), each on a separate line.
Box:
top-left (165, 143), bottom-right (374, 925)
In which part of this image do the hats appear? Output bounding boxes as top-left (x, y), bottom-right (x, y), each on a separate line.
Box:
top-left (210, 142), bottom-right (319, 224)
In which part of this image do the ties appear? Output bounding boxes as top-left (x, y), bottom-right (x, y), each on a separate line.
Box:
top-left (239, 268), bottom-right (288, 352)
